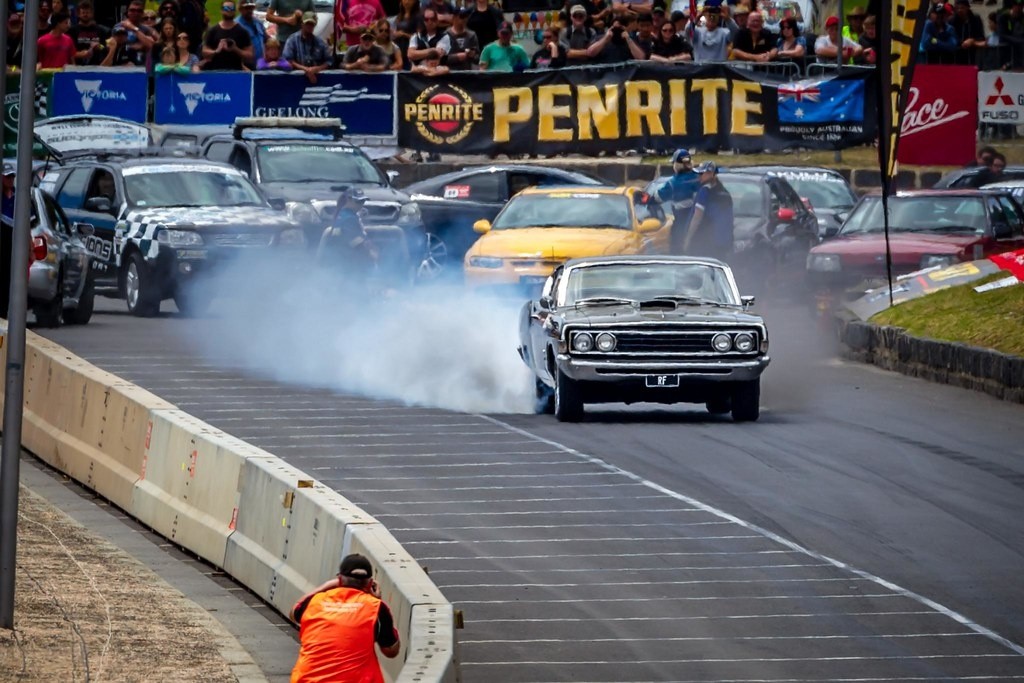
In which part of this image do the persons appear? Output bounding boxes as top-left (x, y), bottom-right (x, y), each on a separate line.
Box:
top-left (289, 554), bottom-right (401, 683)
top-left (0, 0), bottom-right (1023, 318)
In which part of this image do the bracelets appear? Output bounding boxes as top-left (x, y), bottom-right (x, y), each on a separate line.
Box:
top-left (134, 27), bottom-right (139, 33)
top-left (215, 49), bottom-right (217, 54)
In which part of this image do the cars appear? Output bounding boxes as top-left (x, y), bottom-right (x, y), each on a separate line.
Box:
top-left (733, 165), bottom-right (862, 240)
top-left (634, 171), bottom-right (819, 303)
top-left (517, 256), bottom-right (771, 424)
top-left (399, 165), bottom-right (682, 286)
top-left (941, 181), bottom-right (1024, 227)
top-left (804, 187), bottom-right (1024, 297)
top-left (3, 187), bottom-right (96, 327)
top-left (933, 165), bottom-right (1024, 188)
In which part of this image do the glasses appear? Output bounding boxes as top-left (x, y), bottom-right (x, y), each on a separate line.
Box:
top-left (781, 25), bottom-right (791, 30)
top-left (424, 16), bottom-right (436, 22)
top-left (378, 28), bottom-right (389, 33)
top-left (221, 5), bottom-right (236, 11)
top-left (542, 35), bottom-right (552, 39)
top-left (142, 15), bottom-right (156, 21)
top-left (176, 36), bottom-right (188, 40)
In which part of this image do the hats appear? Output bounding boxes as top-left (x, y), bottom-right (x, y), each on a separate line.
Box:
top-left (238, 0), bottom-right (256, 9)
top-left (497, 21), bottom-right (512, 32)
top-left (3, 162), bottom-right (16, 176)
top-left (112, 23), bottom-right (127, 33)
top-left (345, 186), bottom-right (371, 203)
top-left (826, 16), bottom-right (841, 30)
top-left (454, 5), bottom-right (474, 18)
top-left (847, 6), bottom-right (867, 19)
top-left (337, 553), bottom-right (373, 579)
top-left (358, 25), bottom-right (376, 38)
top-left (570, 4), bottom-right (587, 16)
top-left (302, 10), bottom-right (318, 25)
top-left (653, 6), bottom-right (664, 13)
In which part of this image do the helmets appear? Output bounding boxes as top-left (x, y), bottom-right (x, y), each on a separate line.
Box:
top-left (669, 149), bottom-right (692, 163)
top-left (692, 161), bottom-right (719, 174)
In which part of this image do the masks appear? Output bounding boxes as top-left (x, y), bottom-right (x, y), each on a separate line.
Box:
top-left (673, 162), bottom-right (685, 173)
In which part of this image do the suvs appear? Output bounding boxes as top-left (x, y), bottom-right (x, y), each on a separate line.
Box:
top-left (34, 113), bottom-right (307, 316)
top-left (156, 117), bottom-right (427, 284)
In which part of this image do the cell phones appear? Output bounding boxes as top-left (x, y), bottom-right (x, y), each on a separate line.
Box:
top-left (611, 27), bottom-right (624, 32)
top-left (709, 7), bottom-right (721, 13)
